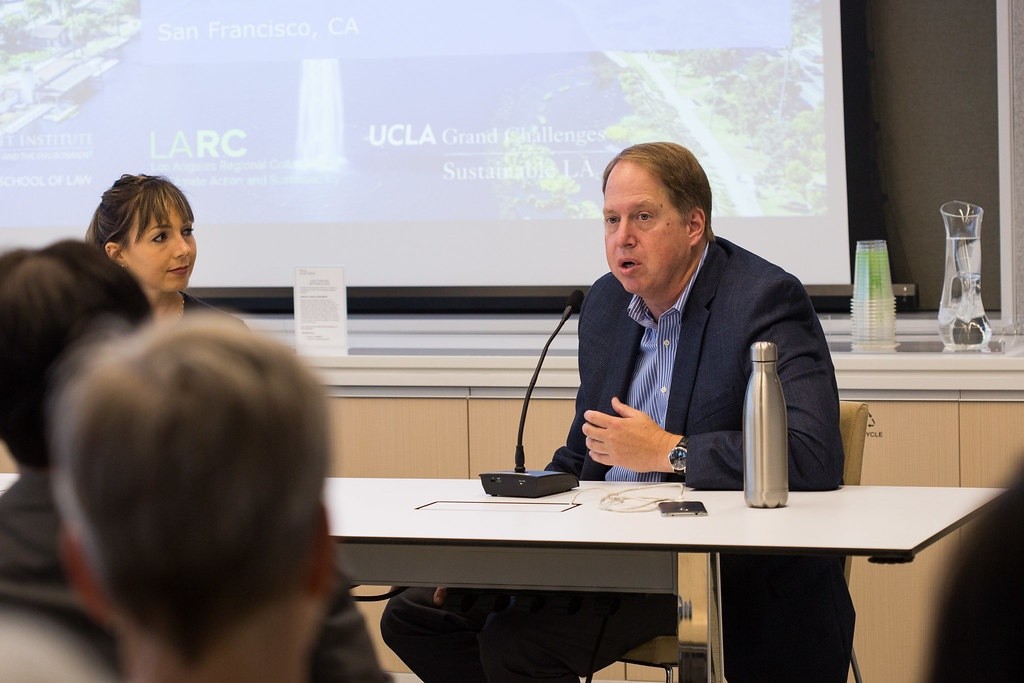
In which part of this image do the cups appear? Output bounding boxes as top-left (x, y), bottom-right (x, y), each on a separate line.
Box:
top-left (849, 240), bottom-right (900, 355)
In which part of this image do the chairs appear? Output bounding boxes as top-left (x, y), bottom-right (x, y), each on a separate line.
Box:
top-left (584, 402), bottom-right (871, 683)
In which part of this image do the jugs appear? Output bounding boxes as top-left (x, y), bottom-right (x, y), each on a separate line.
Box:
top-left (938, 199), bottom-right (992, 351)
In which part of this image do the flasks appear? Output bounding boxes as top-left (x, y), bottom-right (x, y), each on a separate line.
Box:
top-left (743, 342), bottom-right (788, 508)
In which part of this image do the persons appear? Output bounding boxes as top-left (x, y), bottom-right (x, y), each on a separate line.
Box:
top-left (380, 143), bottom-right (855, 683)
top-left (920, 464), bottom-right (1024, 683)
top-left (84, 174), bottom-right (247, 326)
top-left (0, 240), bottom-right (394, 683)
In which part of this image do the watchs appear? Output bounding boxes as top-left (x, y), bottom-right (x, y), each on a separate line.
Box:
top-left (669, 435), bottom-right (688, 478)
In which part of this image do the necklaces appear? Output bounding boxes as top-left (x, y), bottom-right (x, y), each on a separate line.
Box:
top-left (174, 299), bottom-right (185, 321)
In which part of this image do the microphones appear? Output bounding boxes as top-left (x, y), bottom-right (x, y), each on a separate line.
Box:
top-left (479, 289), bottom-right (586, 498)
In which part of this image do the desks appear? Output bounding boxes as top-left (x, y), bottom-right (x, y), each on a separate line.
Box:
top-left (318, 477), bottom-right (1012, 683)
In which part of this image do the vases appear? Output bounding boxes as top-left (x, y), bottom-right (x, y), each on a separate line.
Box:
top-left (936, 199), bottom-right (995, 357)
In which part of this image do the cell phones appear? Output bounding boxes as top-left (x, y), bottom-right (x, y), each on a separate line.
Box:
top-left (658, 501), bottom-right (708, 519)
